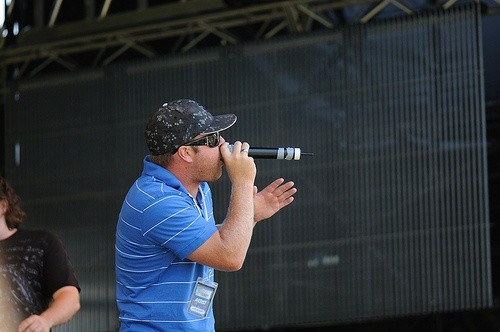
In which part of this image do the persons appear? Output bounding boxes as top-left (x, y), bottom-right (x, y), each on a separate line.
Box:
top-left (114, 99), bottom-right (297, 332)
top-left (0, 178), bottom-right (82, 332)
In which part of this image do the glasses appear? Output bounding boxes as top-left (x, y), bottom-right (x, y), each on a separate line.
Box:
top-left (181, 132), bottom-right (220, 148)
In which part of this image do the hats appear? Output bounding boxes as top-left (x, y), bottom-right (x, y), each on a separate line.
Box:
top-left (145, 99), bottom-right (237, 156)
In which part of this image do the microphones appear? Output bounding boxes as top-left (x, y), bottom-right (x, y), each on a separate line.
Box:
top-left (227, 144), bottom-right (314, 161)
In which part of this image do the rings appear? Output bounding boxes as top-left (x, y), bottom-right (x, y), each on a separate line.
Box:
top-left (241, 149), bottom-right (248, 153)
